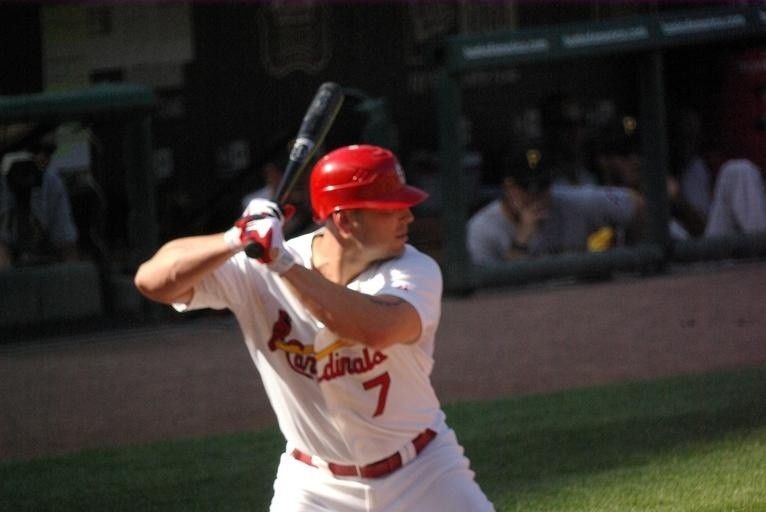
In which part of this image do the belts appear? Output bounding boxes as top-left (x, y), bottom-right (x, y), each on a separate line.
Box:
top-left (292, 428), bottom-right (436, 479)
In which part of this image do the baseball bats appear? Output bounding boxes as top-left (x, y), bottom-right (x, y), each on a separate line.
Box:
top-left (249, 81), bottom-right (344, 257)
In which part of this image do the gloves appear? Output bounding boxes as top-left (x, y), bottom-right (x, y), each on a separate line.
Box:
top-left (225, 198), bottom-right (296, 276)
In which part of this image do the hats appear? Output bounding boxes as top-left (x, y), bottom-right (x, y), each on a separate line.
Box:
top-left (309, 144), bottom-right (429, 224)
top-left (498, 154), bottom-right (561, 186)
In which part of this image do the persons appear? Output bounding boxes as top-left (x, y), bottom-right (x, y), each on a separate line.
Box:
top-left (134, 145), bottom-right (494, 512)
top-left (464, 95), bottom-right (764, 274)
top-left (2, 94), bottom-right (110, 269)
